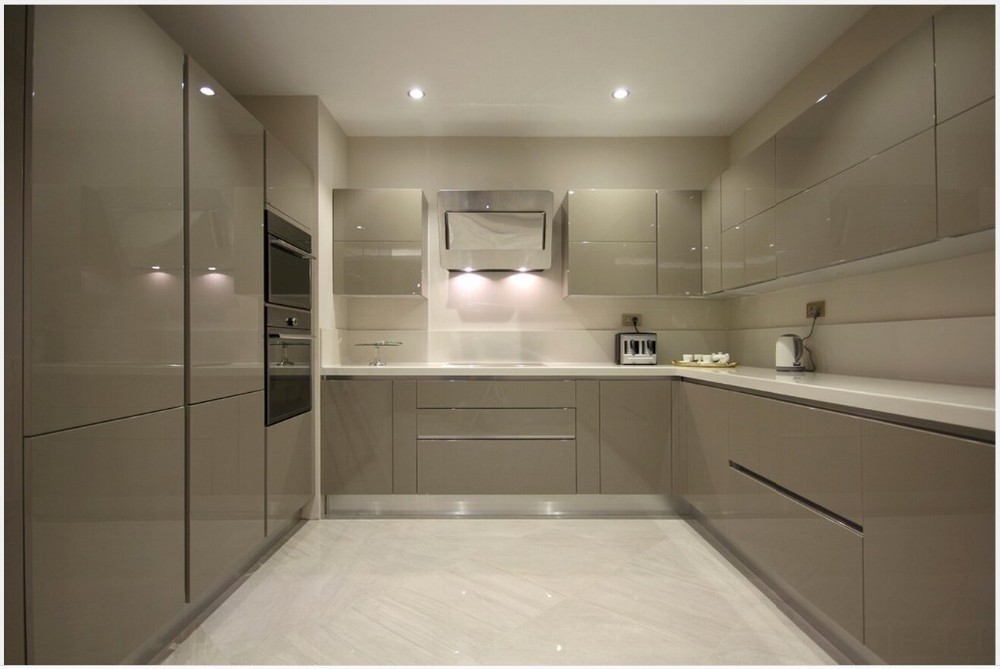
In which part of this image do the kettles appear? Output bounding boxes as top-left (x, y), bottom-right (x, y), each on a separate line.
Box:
top-left (775, 333), bottom-right (806, 372)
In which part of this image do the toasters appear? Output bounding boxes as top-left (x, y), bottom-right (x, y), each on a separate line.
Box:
top-left (615, 330), bottom-right (658, 364)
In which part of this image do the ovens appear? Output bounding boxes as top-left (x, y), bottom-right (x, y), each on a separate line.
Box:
top-left (260, 199), bottom-right (319, 429)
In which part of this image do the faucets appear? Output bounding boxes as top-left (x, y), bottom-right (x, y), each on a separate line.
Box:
top-left (276, 340), bottom-right (295, 368)
top-left (368, 341), bottom-right (389, 366)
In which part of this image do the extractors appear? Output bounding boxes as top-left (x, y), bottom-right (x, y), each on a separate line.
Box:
top-left (436, 188), bottom-right (554, 275)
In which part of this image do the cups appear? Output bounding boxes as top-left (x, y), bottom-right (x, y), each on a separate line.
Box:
top-left (682, 352), bottom-right (729, 365)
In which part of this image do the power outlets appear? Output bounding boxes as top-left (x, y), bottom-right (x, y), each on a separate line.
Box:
top-left (621, 314), bottom-right (642, 326)
top-left (806, 301), bottom-right (826, 319)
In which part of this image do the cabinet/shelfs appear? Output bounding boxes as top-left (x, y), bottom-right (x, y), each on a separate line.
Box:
top-left (730, 389), bottom-right (865, 666)
top-left (321, 375), bottom-right (395, 511)
top-left (865, 412), bottom-right (995, 665)
top-left (933, 4), bottom-right (995, 257)
top-left (776, 21), bottom-right (938, 285)
top-left (561, 189), bottom-right (658, 299)
top-left (416, 378), bottom-right (576, 514)
top-left (680, 377), bottom-right (730, 547)
top-left (600, 377), bottom-right (674, 514)
top-left (25, 5), bottom-right (188, 665)
top-left (332, 187), bottom-right (429, 361)
top-left (185, 52), bottom-right (267, 615)
top-left (702, 173), bottom-right (749, 300)
top-left (656, 189), bottom-right (702, 298)
top-left (720, 135), bottom-right (797, 296)
